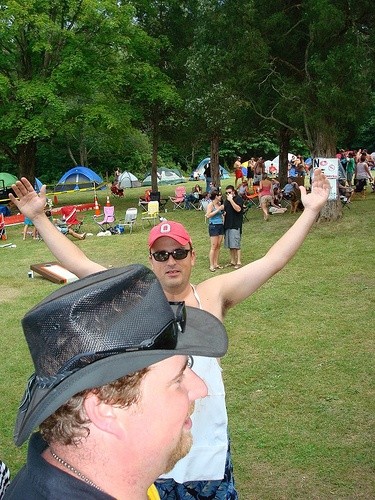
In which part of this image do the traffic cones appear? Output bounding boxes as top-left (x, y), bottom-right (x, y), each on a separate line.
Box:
top-left (106, 195), bottom-right (111, 206)
top-left (95, 197), bottom-right (103, 216)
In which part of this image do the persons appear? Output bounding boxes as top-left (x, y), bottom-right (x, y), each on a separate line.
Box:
top-left (222, 185), bottom-right (244, 269)
top-left (1, 265), bottom-right (229, 500)
top-left (22, 216), bottom-right (38, 241)
top-left (43, 211), bottom-right (87, 240)
top-left (110, 168), bottom-right (125, 198)
top-left (183, 148), bottom-right (375, 222)
top-left (206, 190), bottom-right (226, 271)
top-left (7, 168), bottom-right (332, 500)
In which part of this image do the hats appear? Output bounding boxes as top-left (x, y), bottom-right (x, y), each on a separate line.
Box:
top-left (14, 264), bottom-right (230, 446)
top-left (148, 221), bottom-right (192, 248)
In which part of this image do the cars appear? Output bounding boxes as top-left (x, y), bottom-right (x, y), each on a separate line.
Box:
top-left (336, 151), bottom-right (375, 169)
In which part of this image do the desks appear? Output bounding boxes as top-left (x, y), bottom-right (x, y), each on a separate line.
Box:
top-left (140, 195), bottom-right (170, 212)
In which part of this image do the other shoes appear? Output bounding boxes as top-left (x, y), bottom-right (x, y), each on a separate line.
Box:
top-left (226, 263), bottom-right (236, 267)
top-left (209, 267), bottom-right (216, 272)
top-left (234, 263), bottom-right (241, 268)
top-left (80, 232), bottom-right (86, 240)
top-left (214, 264), bottom-right (223, 269)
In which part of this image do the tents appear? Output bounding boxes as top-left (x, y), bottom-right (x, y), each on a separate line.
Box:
top-left (33, 176), bottom-right (46, 196)
top-left (54, 166), bottom-right (108, 192)
top-left (116, 169), bottom-right (141, 188)
top-left (0, 172), bottom-right (19, 191)
top-left (141, 166), bottom-right (187, 187)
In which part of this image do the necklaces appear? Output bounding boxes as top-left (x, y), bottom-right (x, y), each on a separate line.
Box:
top-left (48, 446), bottom-right (126, 500)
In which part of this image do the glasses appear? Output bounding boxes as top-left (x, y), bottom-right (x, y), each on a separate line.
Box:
top-left (225, 191), bottom-right (232, 194)
top-left (217, 194), bottom-right (222, 196)
top-left (151, 249), bottom-right (192, 262)
top-left (57, 301), bottom-right (187, 376)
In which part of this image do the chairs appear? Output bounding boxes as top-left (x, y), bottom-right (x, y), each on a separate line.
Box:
top-left (170, 187), bottom-right (187, 210)
top-left (187, 187), bottom-right (202, 211)
top-left (242, 203), bottom-right (252, 223)
top-left (282, 197), bottom-right (291, 208)
top-left (141, 201), bottom-right (160, 229)
top-left (93, 205), bottom-right (116, 232)
top-left (339, 187), bottom-right (352, 209)
top-left (118, 208), bottom-right (137, 234)
